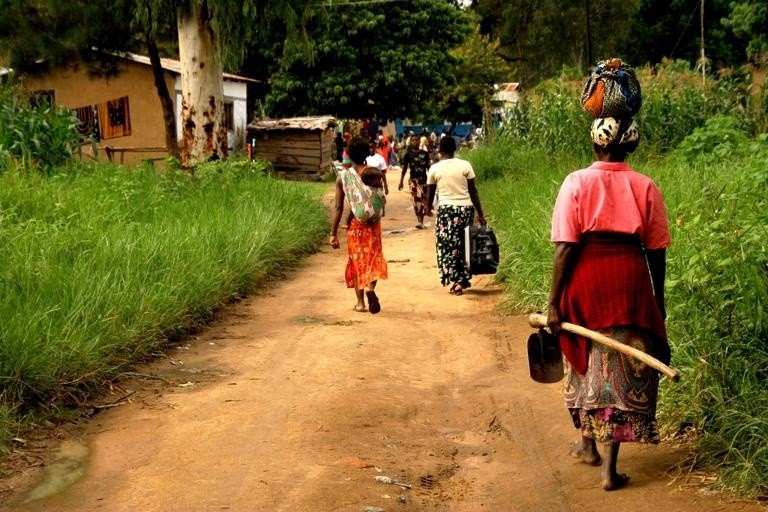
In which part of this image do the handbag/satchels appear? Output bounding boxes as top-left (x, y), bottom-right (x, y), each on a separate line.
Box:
top-left (470, 225), bottom-right (499, 275)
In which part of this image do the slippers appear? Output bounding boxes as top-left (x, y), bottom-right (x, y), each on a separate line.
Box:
top-left (415, 223), bottom-right (427, 229)
top-left (353, 289), bottom-right (381, 314)
top-left (448, 282), bottom-right (471, 296)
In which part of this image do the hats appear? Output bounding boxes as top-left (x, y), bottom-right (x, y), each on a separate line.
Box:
top-left (589, 117), bottom-right (640, 146)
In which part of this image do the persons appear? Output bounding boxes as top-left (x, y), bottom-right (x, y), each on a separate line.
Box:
top-left (399, 135), bottom-right (431, 228)
top-left (547, 116), bottom-right (671, 490)
top-left (330, 137), bottom-right (389, 314)
top-left (426, 137), bottom-right (487, 295)
top-left (340, 167), bottom-right (385, 229)
top-left (333, 128), bottom-right (446, 195)
